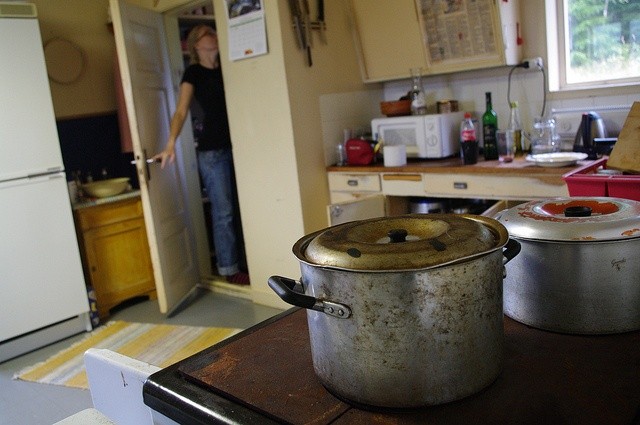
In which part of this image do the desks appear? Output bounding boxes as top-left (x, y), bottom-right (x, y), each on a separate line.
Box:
top-left (142, 306), bottom-right (640, 425)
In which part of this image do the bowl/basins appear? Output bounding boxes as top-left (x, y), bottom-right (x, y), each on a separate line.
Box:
top-left (81, 177), bottom-right (131, 198)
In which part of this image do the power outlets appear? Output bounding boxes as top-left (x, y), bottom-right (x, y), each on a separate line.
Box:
top-left (521, 57), bottom-right (543, 73)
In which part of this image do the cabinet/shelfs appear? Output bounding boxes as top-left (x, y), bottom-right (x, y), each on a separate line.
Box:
top-left (380, 172), bottom-right (570, 201)
top-left (346, 0), bottom-right (523, 85)
top-left (77, 198), bottom-right (157, 320)
top-left (328, 171), bottom-right (383, 227)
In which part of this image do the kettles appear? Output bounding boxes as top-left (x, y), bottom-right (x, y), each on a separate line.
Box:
top-left (572, 111), bottom-right (606, 160)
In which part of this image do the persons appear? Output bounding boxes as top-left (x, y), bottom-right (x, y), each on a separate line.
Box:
top-left (152, 25), bottom-right (248, 285)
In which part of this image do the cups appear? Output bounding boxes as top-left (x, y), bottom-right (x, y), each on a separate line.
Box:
top-left (496, 129), bottom-right (513, 163)
top-left (531, 115), bottom-right (560, 162)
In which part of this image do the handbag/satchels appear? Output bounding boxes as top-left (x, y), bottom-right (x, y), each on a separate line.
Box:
top-left (346, 138), bottom-right (378, 166)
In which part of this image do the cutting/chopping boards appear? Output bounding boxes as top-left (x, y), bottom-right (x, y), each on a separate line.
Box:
top-left (606, 101), bottom-right (640, 174)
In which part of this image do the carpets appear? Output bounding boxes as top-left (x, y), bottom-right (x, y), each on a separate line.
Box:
top-left (13, 320), bottom-right (244, 388)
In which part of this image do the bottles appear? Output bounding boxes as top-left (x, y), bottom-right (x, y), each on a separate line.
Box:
top-left (335, 144), bottom-right (347, 167)
top-left (461, 112), bottom-right (477, 164)
top-left (508, 101), bottom-right (524, 157)
top-left (482, 91), bottom-right (498, 161)
top-left (408, 66), bottom-right (427, 115)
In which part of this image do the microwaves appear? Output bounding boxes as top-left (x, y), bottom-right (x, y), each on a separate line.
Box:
top-left (371, 111), bottom-right (464, 159)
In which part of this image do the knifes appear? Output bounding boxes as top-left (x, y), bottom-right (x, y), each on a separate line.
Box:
top-left (288, 0), bottom-right (305, 50)
top-left (318, 0), bottom-right (328, 45)
top-left (303, 0), bottom-right (313, 48)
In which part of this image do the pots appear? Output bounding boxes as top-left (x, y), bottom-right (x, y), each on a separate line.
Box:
top-left (266, 213), bottom-right (522, 412)
top-left (492, 195), bottom-right (640, 335)
